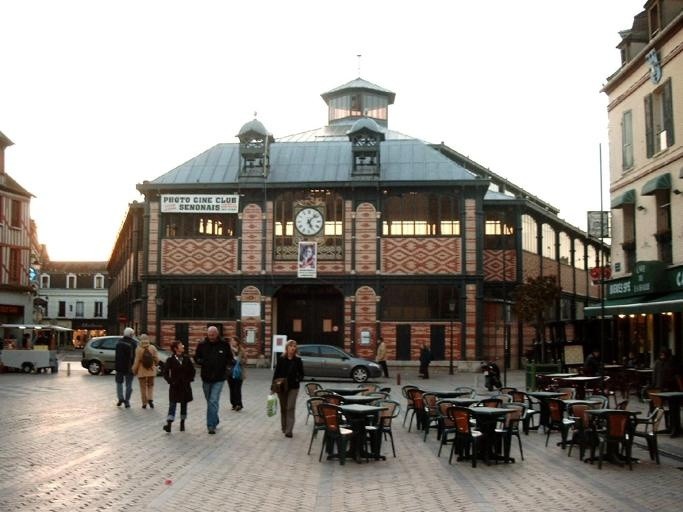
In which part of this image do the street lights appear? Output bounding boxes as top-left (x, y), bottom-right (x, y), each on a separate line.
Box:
top-left (445, 295), bottom-right (457, 376)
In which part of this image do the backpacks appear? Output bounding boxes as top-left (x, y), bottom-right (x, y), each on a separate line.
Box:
top-left (114, 341), bottom-right (134, 371)
top-left (142, 348), bottom-right (154, 370)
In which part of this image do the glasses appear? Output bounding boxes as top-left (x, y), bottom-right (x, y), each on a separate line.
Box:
top-left (177, 344), bottom-right (185, 348)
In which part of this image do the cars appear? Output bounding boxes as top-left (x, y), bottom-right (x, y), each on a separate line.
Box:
top-left (294, 343), bottom-right (382, 381)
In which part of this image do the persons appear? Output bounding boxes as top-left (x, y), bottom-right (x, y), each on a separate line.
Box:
top-left (584, 351), bottom-right (599, 388)
top-left (114, 328), bottom-right (137, 408)
top-left (131, 334), bottom-right (160, 409)
top-left (161, 340), bottom-right (196, 432)
top-left (193, 326), bottom-right (233, 434)
top-left (225, 335), bottom-right (248, 410)
top-left (25, 333), bottom-right (33, 350)
top-left (654, 351), bottom-right (667, 388)
top-left (376, 337), bottom-right (389, 378)
top-left (624, 352), bottom-right (637, 368)
top-left (299, 246), bottom-right (315, 269)
top-left (419, 343), bottom-right (431, 379)
top-left (271, 339), bottom-right (304, 437)
top-left (35, 332), bottom-right (49, 372)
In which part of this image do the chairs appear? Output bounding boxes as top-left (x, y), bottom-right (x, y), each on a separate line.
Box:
top-left (477, 398), bottom-right (504, 408)
top-left (628, 407), bottom-right (665, 465)
top-left (402, 385), bottom-right (421, 430)
top-left (422, 392), bottom-right (442, 442)
top-left (407, 389), bottom-right (426, 433)
top-left (365, 392), bottom-right (388, 441)
top-left (585, 395), bottom-right (607, 435)
top-left (558, 386), bottom-right (578, 399)
top-left (379, 387), bottom-right (393, 391)
top-left (501, 402), bottom-right (528, 462)
top-left (567, 402), bottom-right (597, 461)
top-left (507, 390), bottom-right (541, 437)
top-left (437, 400), bottom-right (466, 458)
top-left (618, 401), bottom-right (631, 409)
top-left (321, 395), bottom-right (345, 405)
top-left (373, 400), bottom-right (401, 458)
top-left (455, 386), bottom-right (476, 399)
top-left (317, 404), bottom-right (356, 467)
top-left (445, 406), bottom-right (491, 468)
top-left (500, 386), bottom-right (517, 394)
top-left (357, 385), bottom-right (381, 396)
top-left (304, 383), bottom-right (323, 427)
top-left (544, 398), bottom-right (577, 450)
top-left (314, 390), bottom-right (333, 399)
top-left (591, 410), bottom-right (637, 473)
top-left (307, 398), bottom-right (332, 457)
top-left (601, 375), bottom-right (619, 410)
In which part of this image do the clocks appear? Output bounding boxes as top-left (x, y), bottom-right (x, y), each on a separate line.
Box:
top-left (293, 200), bottom-right (324, 241)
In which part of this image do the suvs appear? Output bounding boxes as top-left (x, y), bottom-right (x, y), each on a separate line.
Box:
top-left (81, 336), bottom-right (174, 377)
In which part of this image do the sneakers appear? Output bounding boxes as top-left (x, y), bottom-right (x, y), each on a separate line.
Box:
top-left (163, 423), bottom-right (171, 432)
top-left (216, 420), bottom-right (220, 426)
top-left (148, 400), bottom-right (154, 408)
top-left (124, 404), bottom-right (131, 409)
top-left (180, 421), bottom-right (185, 431)
top-left (116, 399), bottom-right (124, 407)
top-left (285, 428), bottom-right (293, 439)
top-left (208, 427), bottom-right (216, 435)
top-left (142, 404), bottom-right (147, 409)
top-left (231, 405), bottom-right (242, 412)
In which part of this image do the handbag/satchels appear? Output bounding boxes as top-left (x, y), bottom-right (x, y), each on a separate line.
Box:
top-left (271, 377), bottom-right (289, 393)
top-left (265, 394), bottom-right (278, 418)
top-left (231, 360), bottom-right (243, 379)
